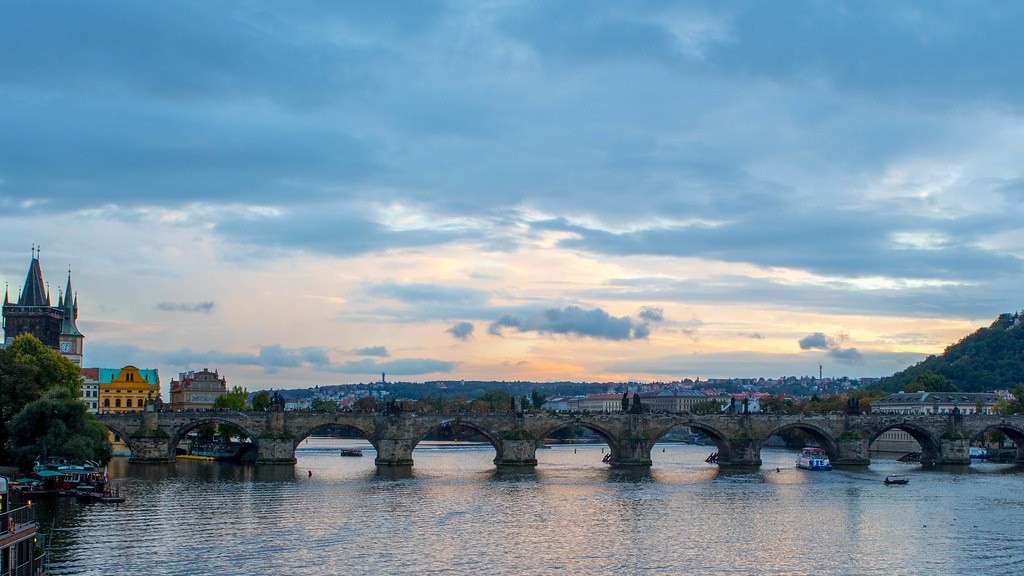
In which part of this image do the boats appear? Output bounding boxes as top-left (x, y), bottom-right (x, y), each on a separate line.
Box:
top-left (10, 454), bottom-right (126, 503)
top-left (0, 476), bottom-right (55, 576)
top-left (795, 447), bottom-right (832, 471)
top-left (176, 454), bottom-right (216, 461)
top-left (970, 454), bottom-right (990, 459)
top-left (884, 474), bottom-right (909, 484)
top-left (340, 449), bottom-right (363, 457)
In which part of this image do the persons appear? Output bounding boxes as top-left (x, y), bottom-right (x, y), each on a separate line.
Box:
top-left (886, 476), bottom-right (889, 481)
top-left (86, 474), bottom-right (93, 496)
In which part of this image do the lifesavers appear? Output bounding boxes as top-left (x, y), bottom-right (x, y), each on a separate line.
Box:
top-left (8, 517), bottom-right (15, 532)
